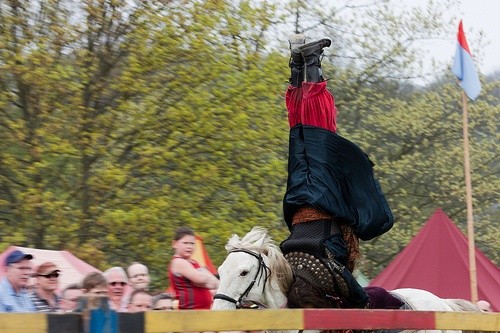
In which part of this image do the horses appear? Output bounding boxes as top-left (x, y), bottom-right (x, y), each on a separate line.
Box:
top-left (209, 226), bottom-right (492, 333)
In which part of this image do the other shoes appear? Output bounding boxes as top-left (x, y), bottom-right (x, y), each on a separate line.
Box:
top-left (289, 33), bottom-right (307, 63)
top-left (299, 38), bottom-right (331, 58)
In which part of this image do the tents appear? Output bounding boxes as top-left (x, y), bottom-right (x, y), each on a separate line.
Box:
top-left (366, 207), bottom-right (500, 313)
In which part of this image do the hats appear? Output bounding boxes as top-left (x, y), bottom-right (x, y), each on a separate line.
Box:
top-left (38, 263), bottom-right (62, 275)
top-left (4, 250), bottom-right (32, 264)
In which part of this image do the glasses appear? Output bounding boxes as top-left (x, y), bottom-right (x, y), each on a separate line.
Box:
top-left (110, 282), bottom-right (128, 286)
top-left (38, 273), bottom-right (60, 278)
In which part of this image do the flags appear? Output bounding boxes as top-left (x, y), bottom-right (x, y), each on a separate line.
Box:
top-left (451, 19), bottom-right (484, 101)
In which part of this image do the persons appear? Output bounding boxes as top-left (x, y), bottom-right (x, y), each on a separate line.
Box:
top-left (57, 283), bottom-right (84, 312)
top-left (0, 248), bottom-right (38, 313)
top-left (126, 289), bottom-right (152, 314)
top-left (72, 270), bottom-right (110, 314)
top-left (121, 263), bottom-right (151, 293)
top-left (286, 31), bottom-right (394, 311)
top-left (167, 226), bottom-right (218, 310)
top-left (25, 261), bottom-right (66, 312)
top-left (101, 265), bottom-right (130, 312)
top-left (150, 292), bottom-right (174, 312)
